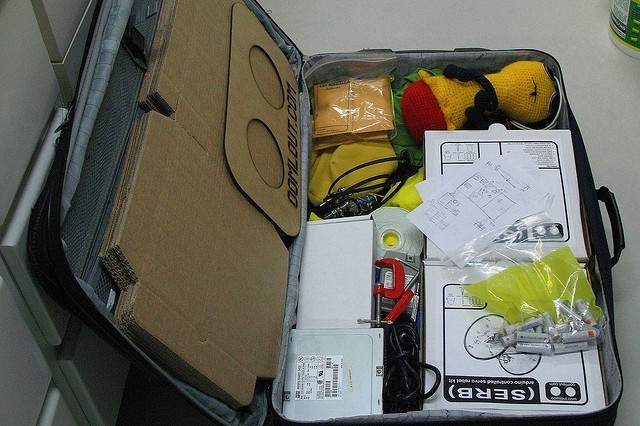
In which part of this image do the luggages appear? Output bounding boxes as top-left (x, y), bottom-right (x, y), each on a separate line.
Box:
top-left (28, 0), bottom-right (625, 426)
